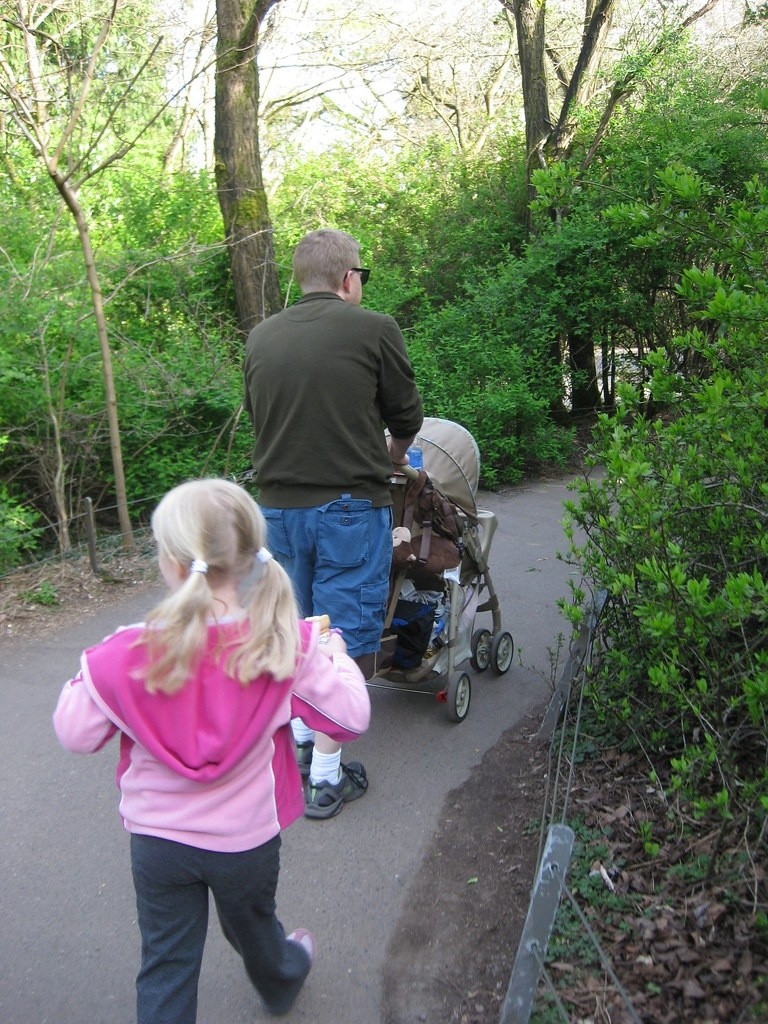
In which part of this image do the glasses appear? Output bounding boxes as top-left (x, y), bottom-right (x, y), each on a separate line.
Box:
top-left (344, 268), bottom-right (371, 286)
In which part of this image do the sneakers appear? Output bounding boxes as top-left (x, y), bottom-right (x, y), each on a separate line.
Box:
top-left (286, 927), bottom-right (316, 963)
top-left (302, 761), bottom-right (369, 818)
top-left (296, 741), bottom-right (316, 774)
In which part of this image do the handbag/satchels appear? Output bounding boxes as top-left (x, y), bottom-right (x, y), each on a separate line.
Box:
top-left (388, 598), bottom-right (438, 672)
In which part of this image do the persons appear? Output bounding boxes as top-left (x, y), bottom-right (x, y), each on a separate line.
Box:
top-left (241, 229), bottom-right (423, 817)
top-left (52, 477), bottom-right (371, 1024)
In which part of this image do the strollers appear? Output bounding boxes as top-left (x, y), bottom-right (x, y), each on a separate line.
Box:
top-left (364, 461), bottom-right (514, 723)
